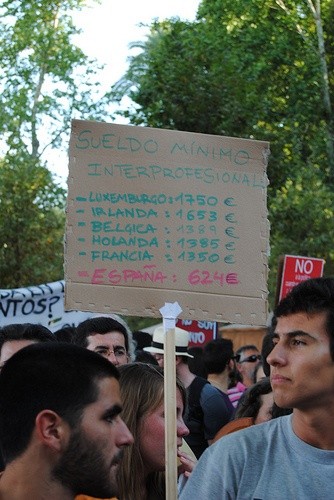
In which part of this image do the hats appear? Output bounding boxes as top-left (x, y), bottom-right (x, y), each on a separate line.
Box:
top-left (143, 327), bottom-right (194, 358)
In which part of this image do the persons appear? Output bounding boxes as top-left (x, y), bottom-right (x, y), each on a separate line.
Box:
top-left (0, 317), bottom-right (294, 500)
top-left (179, 276), bottom-right (334, 500)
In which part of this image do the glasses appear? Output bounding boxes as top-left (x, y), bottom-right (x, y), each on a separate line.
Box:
top-left (239, 355), bottom-right (262, 364)
top-left (93, 349), bottom-right (131, 361)
